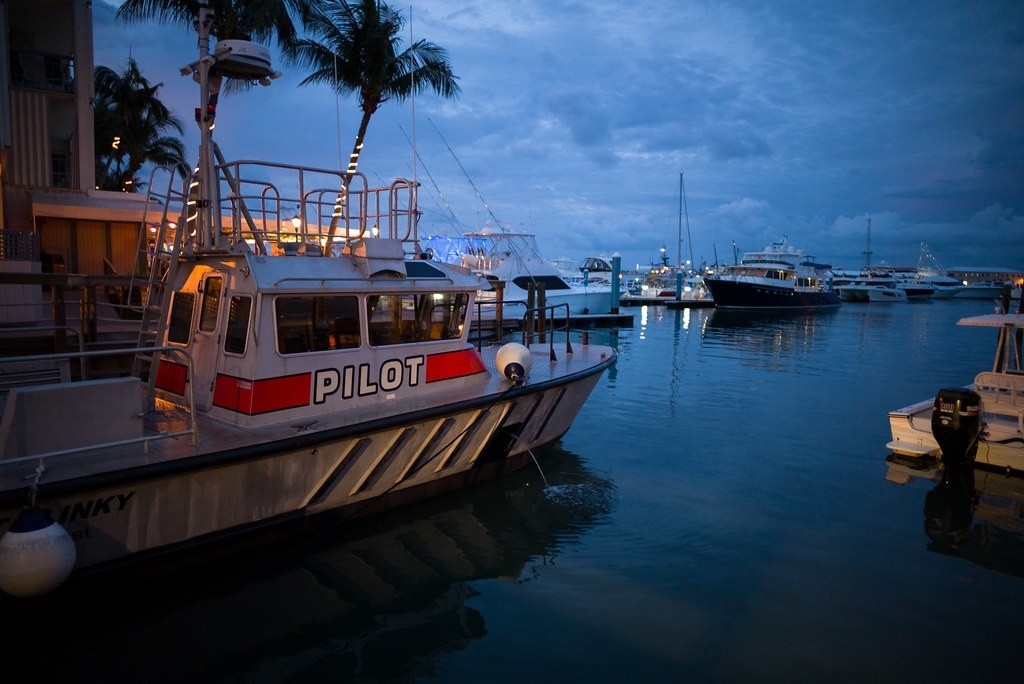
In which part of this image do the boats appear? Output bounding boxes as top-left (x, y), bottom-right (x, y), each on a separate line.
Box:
top-left (1, 4), bottom-right (1024, 339)
top-left (885, 298), bottom-right (1024, 478)
top-left (703, 231), bottom-right (842, 312)
top-left (0, 0), bottom-right (618, 583)
